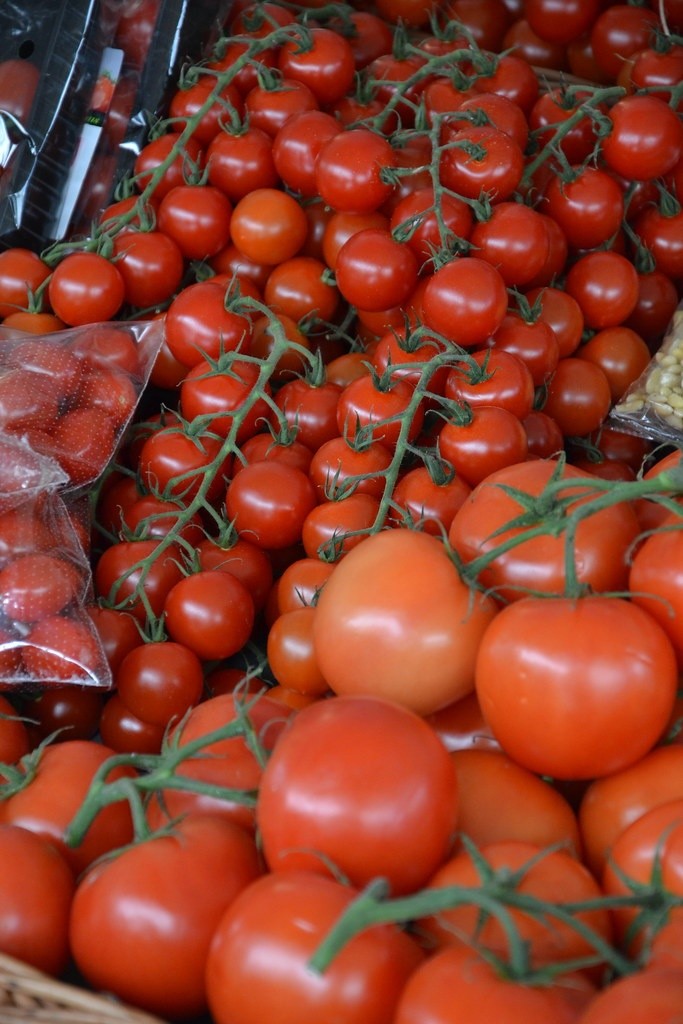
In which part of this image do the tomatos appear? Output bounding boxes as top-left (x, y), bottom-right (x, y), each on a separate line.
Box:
top-left (0, 0), bottom-right (683, 1024)
